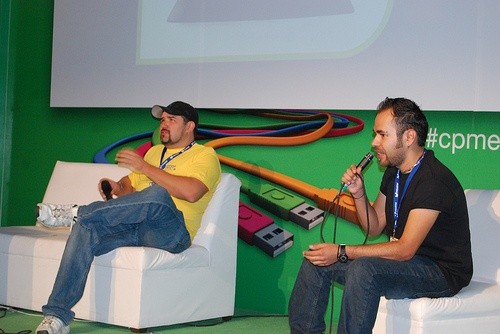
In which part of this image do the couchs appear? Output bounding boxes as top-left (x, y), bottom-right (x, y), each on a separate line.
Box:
top-left (0, 161), bottom-right (243, 333)
top-left (371, 189), bottom-right (500, 334)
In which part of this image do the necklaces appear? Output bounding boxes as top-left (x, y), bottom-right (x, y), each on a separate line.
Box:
top-left (403, 155), bottom-right (424, 175)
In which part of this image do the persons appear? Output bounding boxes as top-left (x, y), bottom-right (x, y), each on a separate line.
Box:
top-left (287, 97), bottom-right (473, 334)
top-left (36, 101), bottom-right (222, 334)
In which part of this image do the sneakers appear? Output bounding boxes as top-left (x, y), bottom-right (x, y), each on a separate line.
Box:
top-left (35, 315), bottom-right (70, 334)
top-left (36, 202), bottom-right (79, 232)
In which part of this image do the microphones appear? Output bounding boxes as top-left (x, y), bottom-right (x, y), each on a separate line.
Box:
top-left (341, 152), bottom-right (375, 187)
top-left (101, 180), bottom-right (113, 201)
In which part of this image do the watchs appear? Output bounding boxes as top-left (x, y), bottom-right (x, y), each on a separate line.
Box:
top-left (339, 244), bottom-right (348, 263)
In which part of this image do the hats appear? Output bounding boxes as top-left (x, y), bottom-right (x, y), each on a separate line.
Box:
top-left (151, 100), bottom-right (199, 126)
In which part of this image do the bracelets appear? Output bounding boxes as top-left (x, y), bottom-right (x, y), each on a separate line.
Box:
top-left (337, 245), bottom-right (340, 261)
top-left (354, 194), bottom-right (365, 199)
top-left (114, 181), bottom-right (123, 195)
